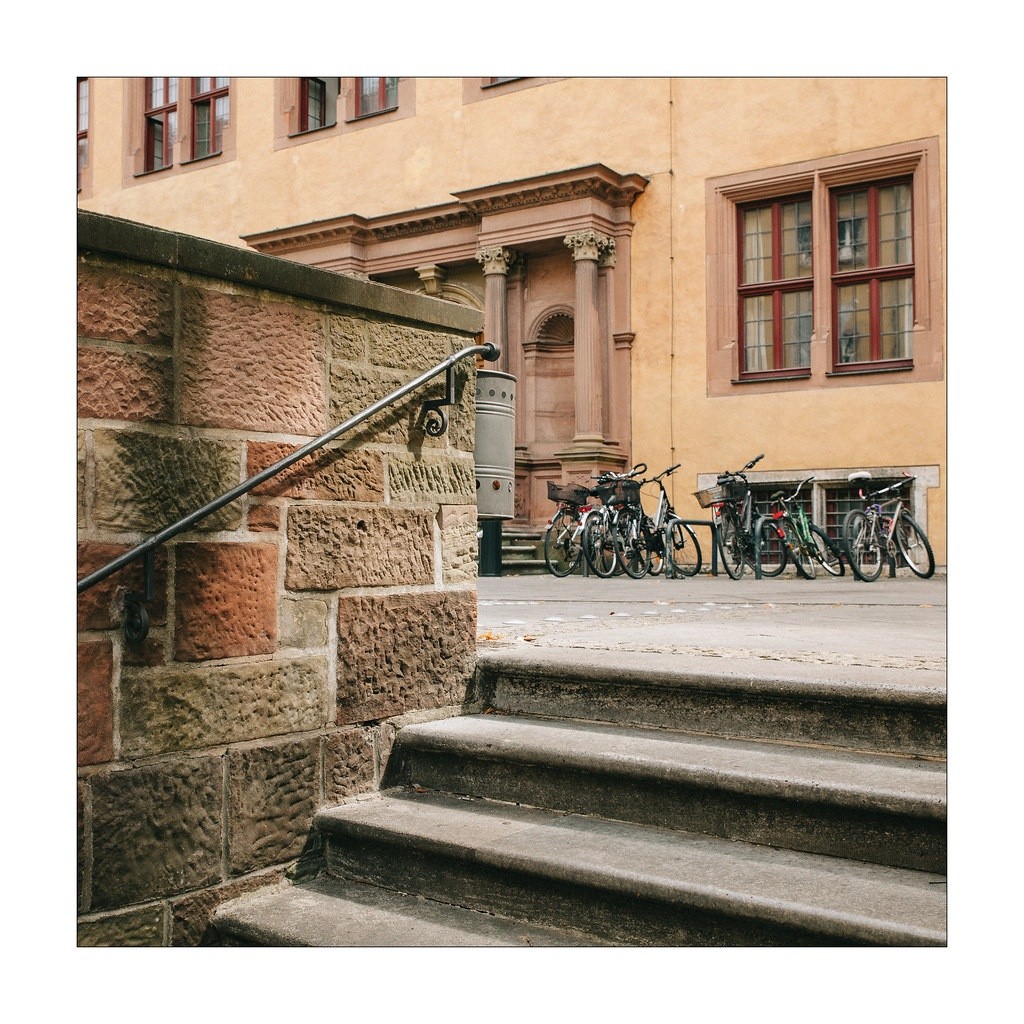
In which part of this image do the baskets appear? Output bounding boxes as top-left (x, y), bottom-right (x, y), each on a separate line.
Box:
top-left (691, 482), bottom-right (729, 509)
top-left (595, 478), bottom-right (641, 507)
top-left (546, 480), bottom-right (590, 506)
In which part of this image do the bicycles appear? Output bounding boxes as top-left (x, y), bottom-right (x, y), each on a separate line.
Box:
top-left (770, 476), bottom-right (845, 580)
top-left (841, 471), bottom-right (936, 582)
top-left (543, 475), bottom-right (620, 578)
top-left (610, 463), bottom-right (702, 579)
top-left (578, 463), bottom-right (665, 578)
top-left (690, 454), bottom-right (788, 580)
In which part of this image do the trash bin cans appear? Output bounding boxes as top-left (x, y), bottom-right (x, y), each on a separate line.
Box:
top-left (473, 368), bottom-right (517, 520)
